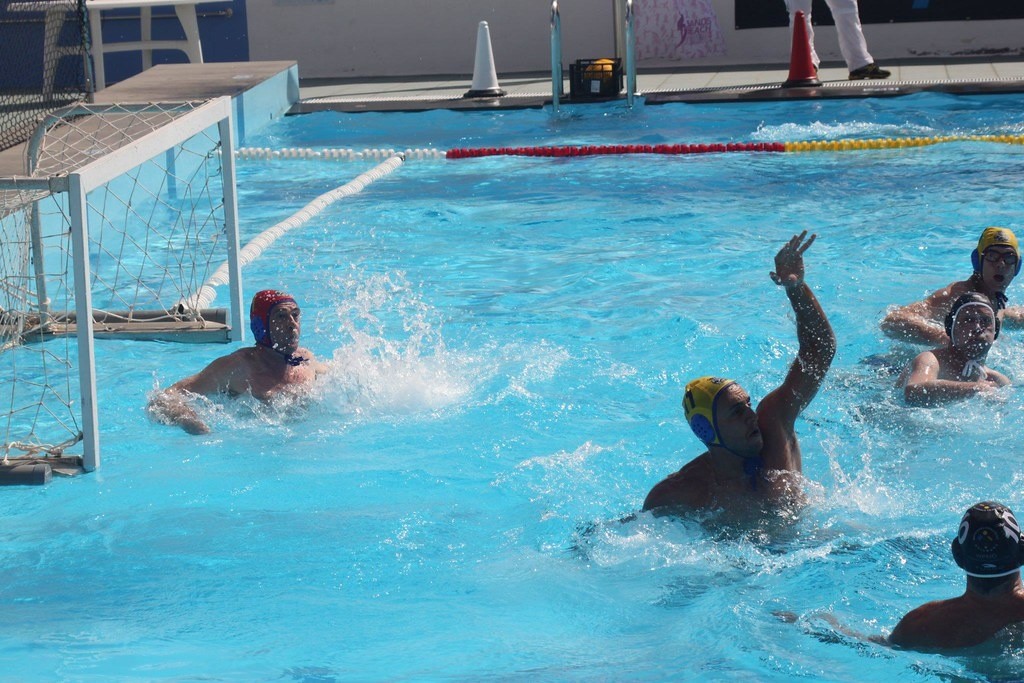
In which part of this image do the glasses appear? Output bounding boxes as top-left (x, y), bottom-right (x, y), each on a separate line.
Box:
top-left (983, 250), bottom-right (1017, 264)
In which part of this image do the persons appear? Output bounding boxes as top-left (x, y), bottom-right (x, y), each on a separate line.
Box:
top-left (778, 500), bottom-right (1024, 650)
top-left (146, 289), bottom-right (328, 435)
top-left (865, 226), bottom-right (1024, 408)
top-left (785, 0), bottom-right (891, 81)
top-left (643, 229), bottom-right (838, 509)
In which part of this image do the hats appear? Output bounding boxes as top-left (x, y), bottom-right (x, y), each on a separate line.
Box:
top-left (683, 377), bottom-right (736, 447)
top-left (952, 502), bottom-right (1024, 578)
top-left (971, 227), bottom-right (1022, 280)
top-left (945, 292), bottom-right (1001, 348)
top-left (251, 290), bottom-right (298, 348)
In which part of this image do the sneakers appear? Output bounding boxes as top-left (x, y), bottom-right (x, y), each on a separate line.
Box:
top-left (849, 63), bottom-right (890, 80)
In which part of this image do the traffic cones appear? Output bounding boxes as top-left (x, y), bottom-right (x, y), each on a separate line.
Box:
top-left (461, 21), bottom-right (508, 97)
top-left (780, 9), bottom-right (824, 88)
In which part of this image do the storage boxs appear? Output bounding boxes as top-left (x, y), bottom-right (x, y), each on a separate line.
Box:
top-left (569, 58), bottom-right (624, 98)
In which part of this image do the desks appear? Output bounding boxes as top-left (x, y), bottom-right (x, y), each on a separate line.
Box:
top-left (6, 1), bottom-right (232, 102)
